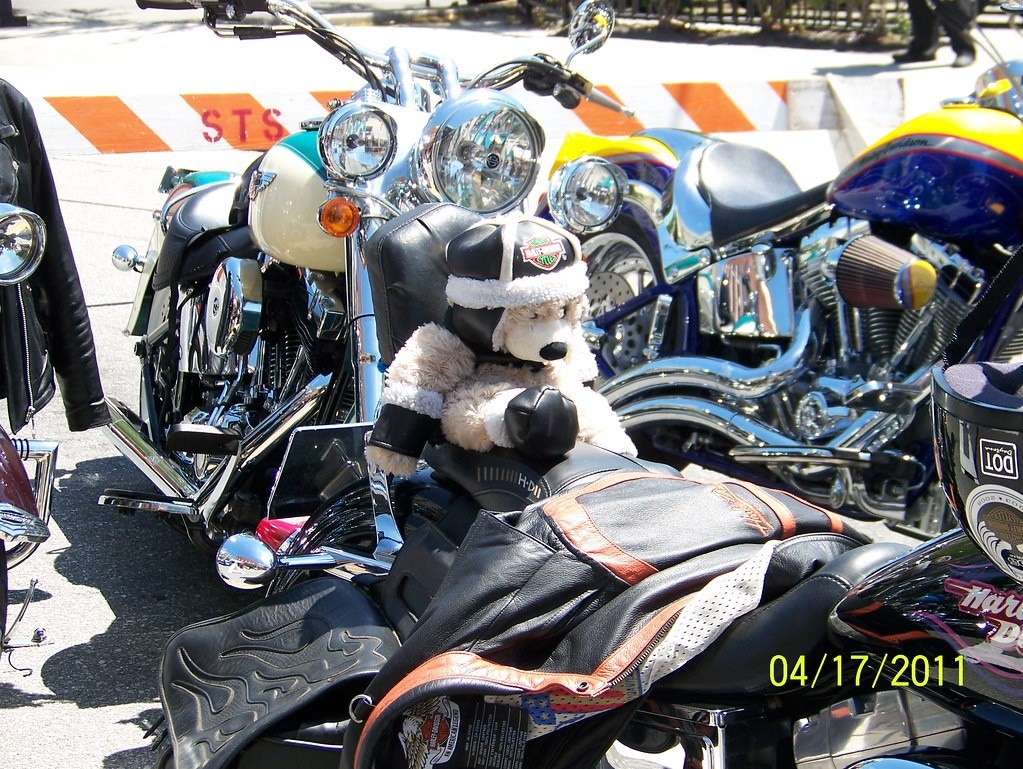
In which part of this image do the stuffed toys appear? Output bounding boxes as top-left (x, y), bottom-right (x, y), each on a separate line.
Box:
top-left (365, 215), bottom-right (637, 476)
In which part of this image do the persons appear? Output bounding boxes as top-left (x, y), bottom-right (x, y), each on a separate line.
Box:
top-left (892, 0), bottom-right (975, 68)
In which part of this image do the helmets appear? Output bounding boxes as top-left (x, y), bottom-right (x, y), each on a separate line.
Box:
top-left (928, 357), bottom-right (1023, 587)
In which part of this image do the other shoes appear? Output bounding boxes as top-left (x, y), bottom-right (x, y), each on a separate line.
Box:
top-left (952, 44), bottom-right (976, 68)
top-left (893, 43), bottom-right (934, 63)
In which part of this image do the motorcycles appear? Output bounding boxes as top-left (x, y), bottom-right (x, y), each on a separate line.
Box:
top-left (0, 0), bottom-right (1023, 767)
